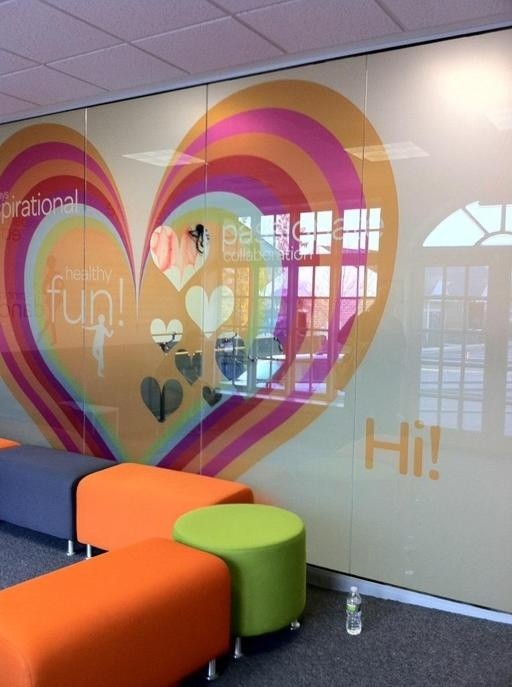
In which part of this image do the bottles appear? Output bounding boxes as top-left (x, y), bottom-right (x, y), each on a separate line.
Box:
top-left (345, 585), bottom-right (363, 636)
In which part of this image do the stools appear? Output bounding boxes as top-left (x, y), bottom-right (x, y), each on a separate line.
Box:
top-left (173, 500), bottom-right (308, 657)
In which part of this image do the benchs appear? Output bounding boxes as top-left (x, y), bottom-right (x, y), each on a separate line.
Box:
top-left (76, 462), bottom-right (253, 558)
top-left (0, 536), bottom-right (232, 686)
top-left (0, 443), bottom-right (118, 557)
